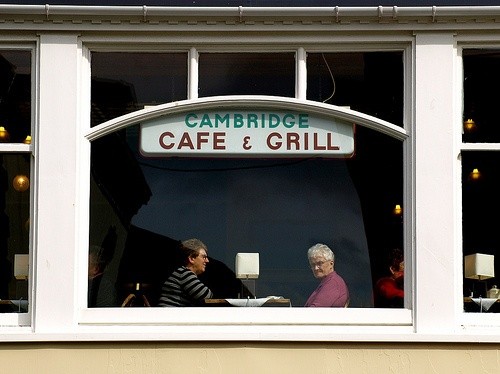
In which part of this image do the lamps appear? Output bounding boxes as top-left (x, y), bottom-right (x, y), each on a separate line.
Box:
top-left (235, 253), bottom-right (259, 298)
top-left (14, 254), bottom-right (29, 300)
top-left (464, 253), bottom-right (495, 298)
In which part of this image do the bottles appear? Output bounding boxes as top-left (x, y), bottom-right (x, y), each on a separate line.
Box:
top-left (490, 286), bottom-right (500, 299)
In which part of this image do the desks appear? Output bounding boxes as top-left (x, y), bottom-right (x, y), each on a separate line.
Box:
top-left (0, 300), bottom-right (28, 313)
top-left (464, 297), bottom-right (500, 311)
top-left (204, 298), bottom-right (290, 304)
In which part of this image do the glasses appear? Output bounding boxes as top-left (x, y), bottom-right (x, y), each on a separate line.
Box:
top-left (311, 259), bottom-right (330, 266)
top-left (202, 255), bottom-right (207, 260)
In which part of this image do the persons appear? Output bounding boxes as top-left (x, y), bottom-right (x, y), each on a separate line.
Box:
top-left (156, 238), bottom-right (214, 307)
top-left (88, 251), bottom-right (115, 308)
top-left (374, 251), bottom-right (403, 309)
top-left (303, 243), bottom-right (350, 307)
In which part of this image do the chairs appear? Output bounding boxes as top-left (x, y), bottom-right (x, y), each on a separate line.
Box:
top-left (121, 294), bottom-right (351, 307)
